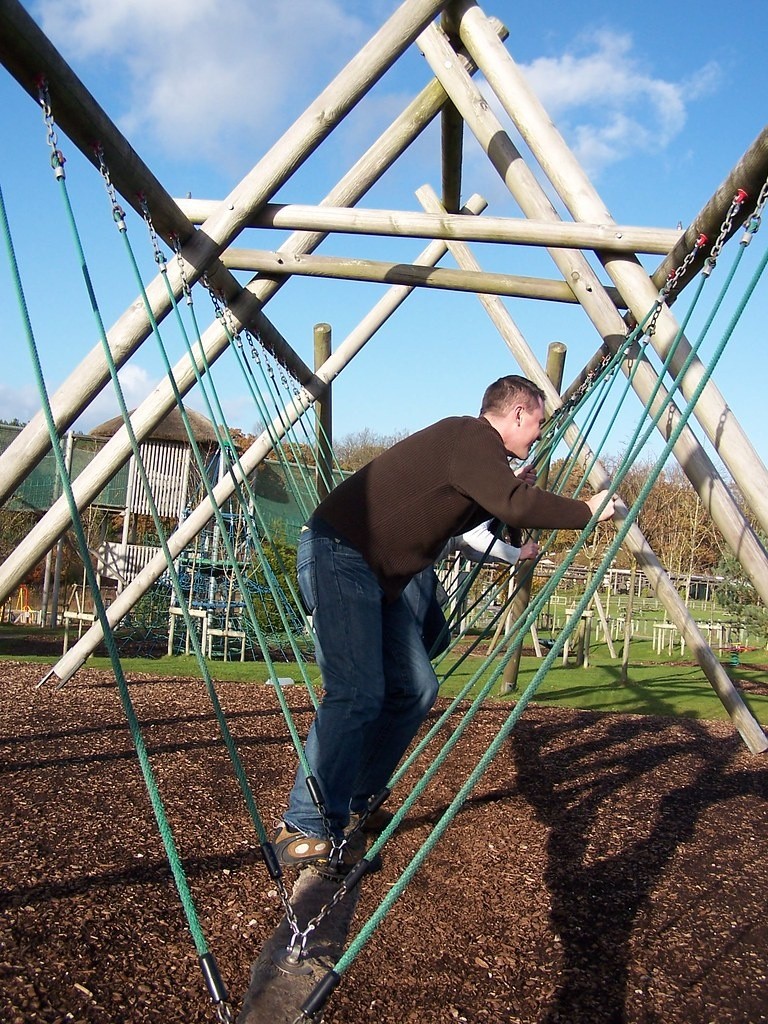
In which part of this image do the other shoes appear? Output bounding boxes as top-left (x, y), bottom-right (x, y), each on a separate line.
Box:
top-left (343, 807), bottom-right (398, 832)
top-left (272, 823), bottom-right (331, 865)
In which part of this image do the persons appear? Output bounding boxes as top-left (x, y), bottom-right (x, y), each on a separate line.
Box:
top-left (402, 513), bottom-right (540, 661)
top-left (272, 375), bottom-right (620, 881)
